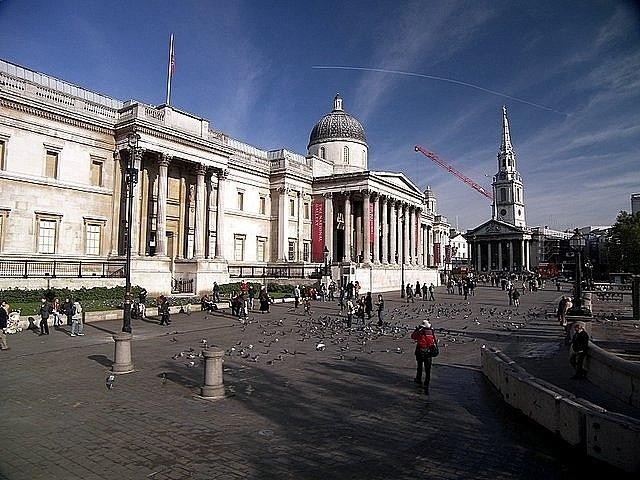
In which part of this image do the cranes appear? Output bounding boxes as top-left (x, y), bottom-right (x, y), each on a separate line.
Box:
top-left (414, 144), bottom-right (495, 202)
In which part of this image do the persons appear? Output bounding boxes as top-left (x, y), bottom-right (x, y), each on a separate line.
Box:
top-left (568, 324), bottom-right (589, 370)
top-left (406, 274), bottom-right (574, 326)
top-left (0, 297), bottom-right (84, 351)
top-left (129, 279), bottom-right (384, 328)
top-left (411, 319), bottom-right (437, 396)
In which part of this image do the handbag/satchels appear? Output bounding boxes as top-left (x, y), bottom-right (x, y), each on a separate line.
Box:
top-left (424, 345), bottom-right (439, 357)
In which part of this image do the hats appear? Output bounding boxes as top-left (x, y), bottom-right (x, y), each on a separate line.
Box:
top-left (420, 320), bottom-right (432, 329)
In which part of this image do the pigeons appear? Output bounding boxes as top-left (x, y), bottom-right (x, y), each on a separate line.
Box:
top-left (107, 372), bottom-right (115, 391)
top-left (160, 372), bottom-right (167, 384)
top-left (593, 311), bottom-right (620, 323)
top-left (168, 303), bottom-right (558, 373)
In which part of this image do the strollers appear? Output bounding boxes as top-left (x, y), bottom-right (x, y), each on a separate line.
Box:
top-left (131, 301), bottom-right (146, 320)
top-left (3, 309), bottom-right (23, 334)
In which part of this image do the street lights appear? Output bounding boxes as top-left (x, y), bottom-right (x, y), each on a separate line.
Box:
top-left (121, 124), bottom-right (143, 332)
top-left (569, 227), bottom-right (585, 308)
top-left (322, 245), bottom-right (329, 276)
